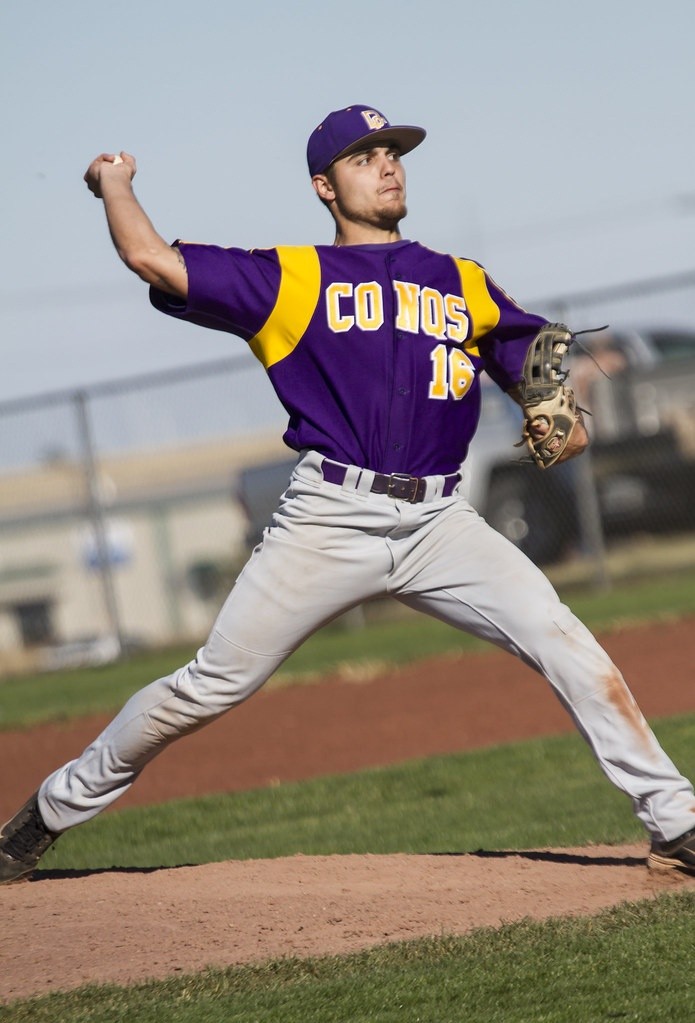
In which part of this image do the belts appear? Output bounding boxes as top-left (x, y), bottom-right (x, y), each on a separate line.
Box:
top-left (296, 450), bottom-right (462, 505)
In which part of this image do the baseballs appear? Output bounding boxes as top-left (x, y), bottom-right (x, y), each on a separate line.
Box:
top-left (113, 154), bottom-right (123, 166)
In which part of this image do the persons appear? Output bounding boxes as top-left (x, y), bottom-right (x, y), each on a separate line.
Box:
top-left (0, 105), bottom-right (694, 883)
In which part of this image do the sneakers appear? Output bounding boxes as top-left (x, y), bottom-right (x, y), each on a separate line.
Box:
top-left (646, 827), bottom-right (695, 873)
top-left (1, 787), bottom-right (65, 884)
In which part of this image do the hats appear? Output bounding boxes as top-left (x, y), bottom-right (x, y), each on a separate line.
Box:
top-left (307, 103), bottom-right (426, 178)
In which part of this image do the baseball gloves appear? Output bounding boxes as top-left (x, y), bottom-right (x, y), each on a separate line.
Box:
top-left (519, 323), bottom-right (577, 468)
